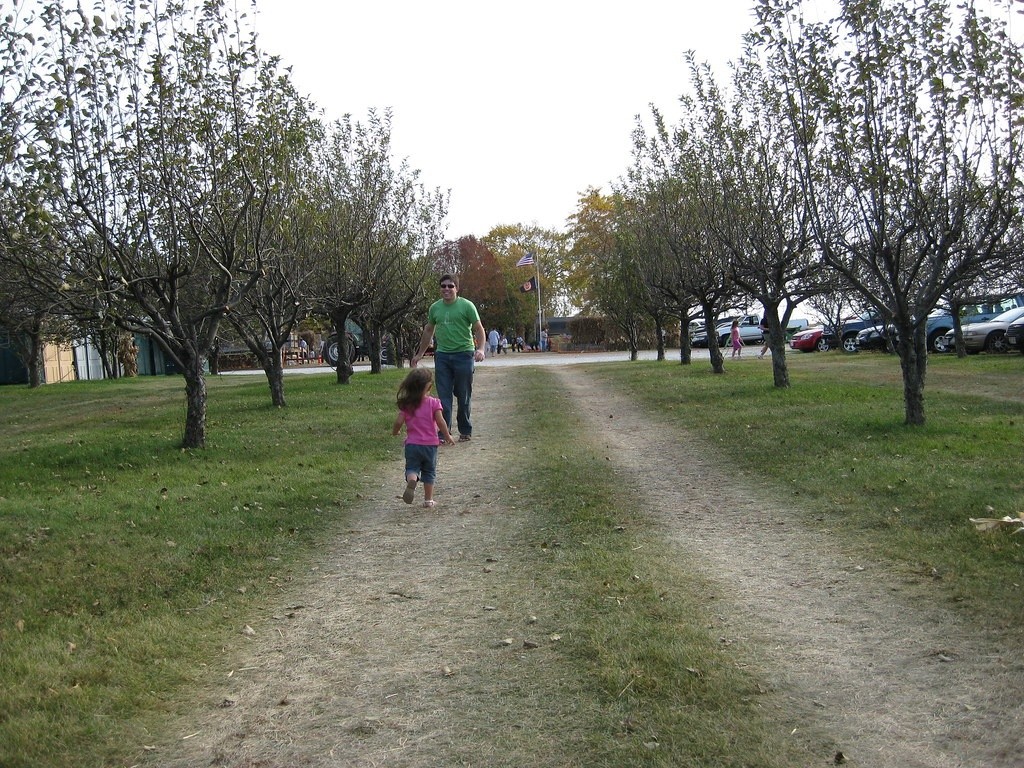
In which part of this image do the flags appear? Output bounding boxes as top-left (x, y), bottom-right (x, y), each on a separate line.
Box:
top-left (515, 252), bottom-right (534, 267)
top-left (519, 276), bottom-right (537, 294)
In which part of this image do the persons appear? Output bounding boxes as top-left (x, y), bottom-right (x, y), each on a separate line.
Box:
top-left (309, 347), bottom-right (315, 363)
top-left (409, 275), bottom-right (487, 443)
top-left (392, 366), bottom-right (456, 508)
top-left (730, 321), bottom-right (743, 358)
top-left (496, 332), bottom-right (524, 354)
top-left (488, 326), bottom-right (500, 357)
top-left (541, 328), bottom-right (552, 351)
top-left (758, 310), bottom-right (772, 359)
top-left (299, 338), bottom-right (308, 358)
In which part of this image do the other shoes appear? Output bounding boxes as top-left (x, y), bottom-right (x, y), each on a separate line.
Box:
top-left (424, 499), bottom-right (437, 508)
top-left (403, 480), bottom-right (417, 504)
top-left (758, 355), bottom-right (763, 360)
top-left (458, 435), bottom-right (471, 442)
top-left (439, 439), bottom-right (446, 445)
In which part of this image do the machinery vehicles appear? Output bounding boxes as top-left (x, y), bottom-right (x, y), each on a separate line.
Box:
top-left (321, 318), bottom-right (388, 367)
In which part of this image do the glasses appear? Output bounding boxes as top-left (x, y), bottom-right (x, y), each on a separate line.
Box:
top-left (441, 283), bottom-right (454, 289)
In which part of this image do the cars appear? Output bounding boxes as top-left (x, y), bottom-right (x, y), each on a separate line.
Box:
top-left (944, 305), bottom-right (1024, 353)
top-left (1004, 311), bottom-right (1024, 357)
top-left (691, 291), bottom-right (1024, 353)
